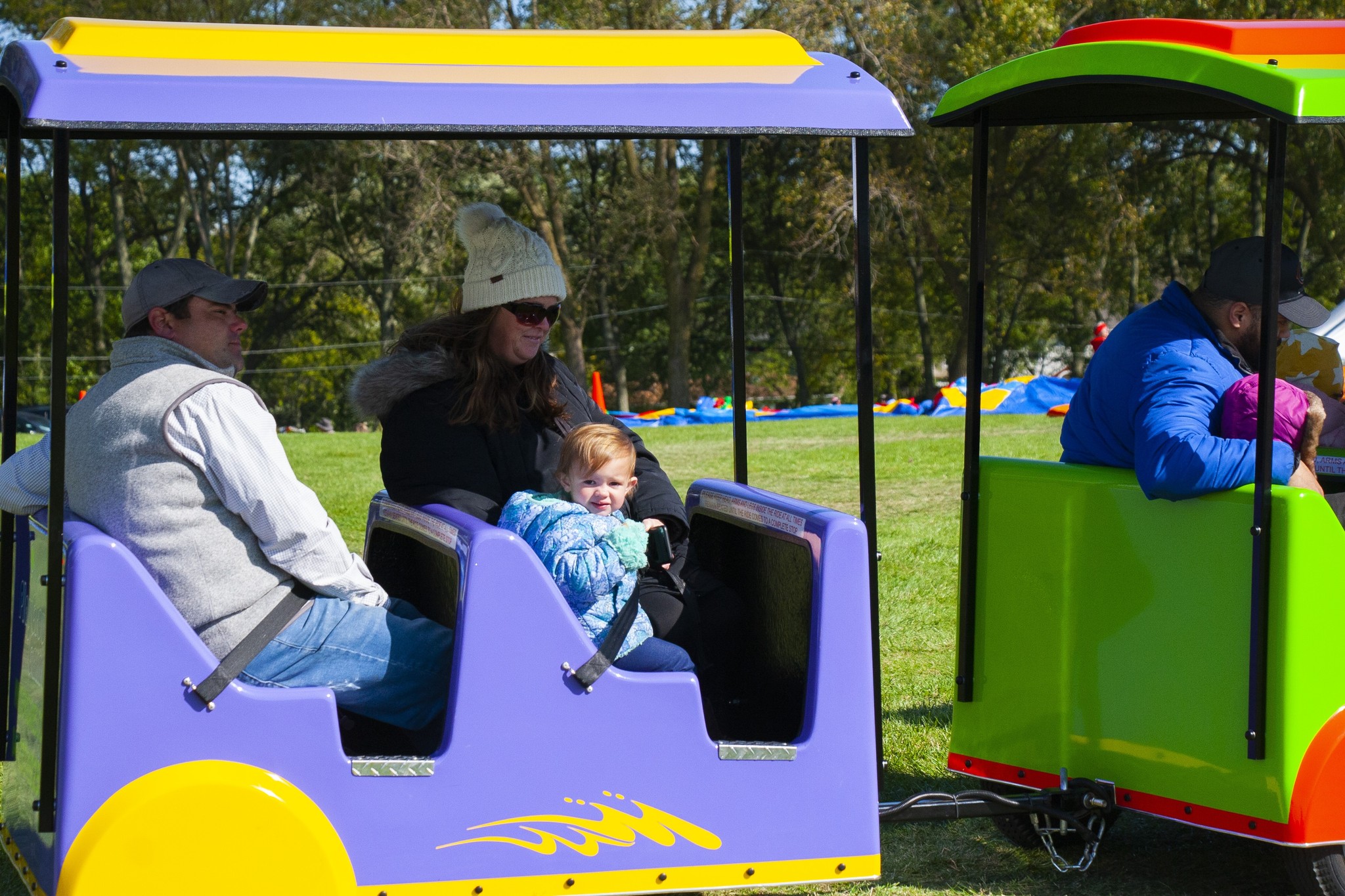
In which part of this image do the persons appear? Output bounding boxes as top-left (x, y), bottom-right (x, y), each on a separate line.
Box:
top-left (496, 421), bottom-right (695, 676)
top-left (1214, 373), bottom-right (1326, 479)
top-left (0, 258), bottom-right (462, 734)
top-left (345, 200), bottom-right (751, 724)
top-left (1057, 234), bottom-right (1327, 500)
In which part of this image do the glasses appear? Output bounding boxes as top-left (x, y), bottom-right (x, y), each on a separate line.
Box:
top-left (501, 302), bottom-right (561, 326)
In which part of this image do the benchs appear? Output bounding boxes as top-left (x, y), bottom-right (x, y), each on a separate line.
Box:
top-left (955, 454), bottom-right (1345, 690)
top-left (9, 509), bottom-right (345, 759)
top-left (363, 491), bottom-right (710, 756)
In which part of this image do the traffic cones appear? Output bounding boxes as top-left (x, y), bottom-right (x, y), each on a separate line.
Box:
top-left (592, 371), bottom-right (606, 415)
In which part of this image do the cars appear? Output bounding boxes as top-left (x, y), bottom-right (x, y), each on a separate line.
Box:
top-left (15, 410), bottom-right (51, 434)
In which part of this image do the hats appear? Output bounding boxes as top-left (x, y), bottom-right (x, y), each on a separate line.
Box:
top-left (454, 202), bottom-right (566, 314)
top-left (1204, 235), bottom-right (1331, 329)
top-left (121, 258), bottom-right (268, 334)
